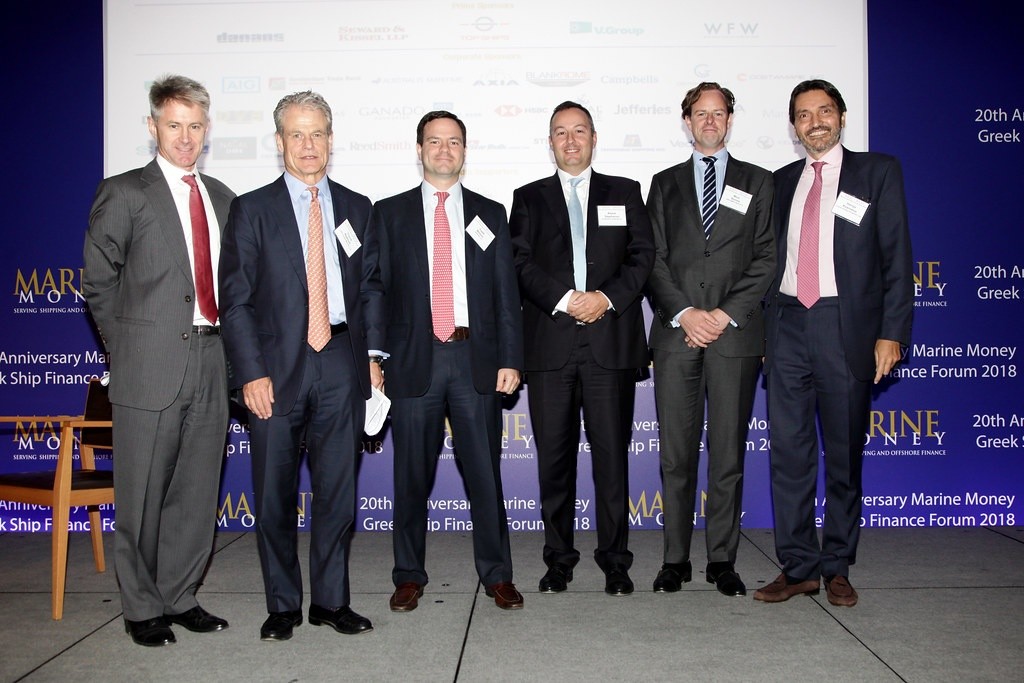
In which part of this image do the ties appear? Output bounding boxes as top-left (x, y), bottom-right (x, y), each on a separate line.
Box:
top-left (700, 155), bottom-right (720, 242)
top-left (305, 186), bottom-right (331, 352)
top-left (181, 174), bottom-right (218, 326)
top-left (797, 162), bottom-right (828, 309)
top-left (564, 177), bottom-right (586, 295)
top-left (431, 191), bottom-right (456, 343)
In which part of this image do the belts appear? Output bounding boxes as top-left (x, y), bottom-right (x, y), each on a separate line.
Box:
top-left (432, 327), bottom-right (469, 341)
top-left (330, 323), bottom-right (348, 335)
top-left (192, 325), bottom-right (221, 336)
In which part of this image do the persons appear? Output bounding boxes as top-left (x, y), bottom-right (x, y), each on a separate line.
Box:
top-left (374, 111), bottom-right (524, 612)
top-left (645, 83), bottom-right (779, 597)
top-left (219, 90), bottom-right (374, 641)
top-left (83, 75), bottom-right (238, 648)
top-left (509, 101), bottom-right (651, 596)
top-left (754, 80), bottom-right (915, 607)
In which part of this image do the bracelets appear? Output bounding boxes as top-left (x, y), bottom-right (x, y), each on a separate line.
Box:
top-left (370, 357), bottom-right (383, 362)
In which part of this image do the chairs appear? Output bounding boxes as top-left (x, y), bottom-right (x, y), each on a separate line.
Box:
top-left (0, 378), bottom-right (115, 621)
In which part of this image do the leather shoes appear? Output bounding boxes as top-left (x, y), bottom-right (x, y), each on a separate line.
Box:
top-left (260, 609), bottom-right (302, 641)
top-left (308, 602), bottom-right (373, 634)
top-left (706, 562), bottom-right (747, 596)
top-left (124, 616), bottom-right (176, 647)
top-left (163, 605), bottom-right (228, 633)
top-left (752, 574), bottom-right (821, 603)
top-left (485, 582), bottom-right (523, 610)
top-left (605, 570), bottom-right (634, 596)
top-left (390, 581), bottom-right (423, 612)
top-left (823, 575), bottom-right (858, 608)
top-left (653, 560), bottom-right (693, 593)
top-left (539, 569), bottom-right (573, 594)
